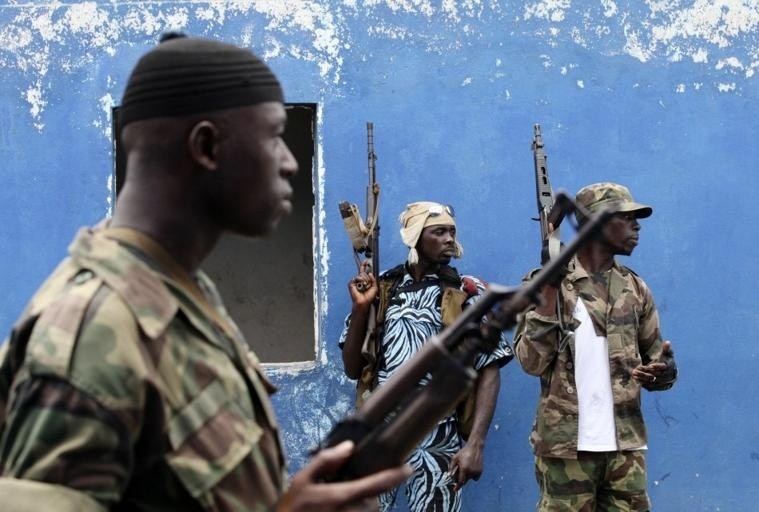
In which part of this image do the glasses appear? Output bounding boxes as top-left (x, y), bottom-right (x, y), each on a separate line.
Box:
top-left (428, 204), bottom-right (455, 217)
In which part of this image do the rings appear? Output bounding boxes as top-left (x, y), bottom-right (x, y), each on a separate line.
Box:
top-left (650, 376), bottom-right (656, 382)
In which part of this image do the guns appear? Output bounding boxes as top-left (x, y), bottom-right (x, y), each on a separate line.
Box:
top-left (304, 192), bottom-right (612, 486)
top-left (529, 122), bottom-right (566, 265)
top-left (339, 121), bottom-right (382, 365)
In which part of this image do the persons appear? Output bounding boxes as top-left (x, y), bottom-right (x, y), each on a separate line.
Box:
top-left (336, 199), bottom-right (515, 512)
top-left (0, 38), bottom-right (415, 512)
top-left (512, 181), bottom-right (680, 512)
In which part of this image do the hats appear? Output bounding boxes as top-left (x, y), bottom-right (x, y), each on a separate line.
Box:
top-left (115, 37), bottom-right (283, 131)
top-left (574, 182), bottom-right (653, 226)
top-left (399, 200), bottom-right (464, 268)
